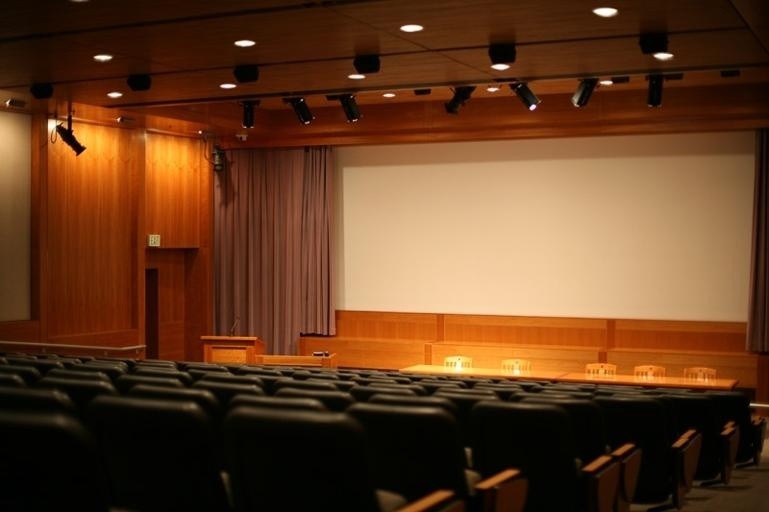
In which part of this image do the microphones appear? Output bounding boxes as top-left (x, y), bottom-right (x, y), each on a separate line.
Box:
top-left (230, 319), bottom-right (240, 332)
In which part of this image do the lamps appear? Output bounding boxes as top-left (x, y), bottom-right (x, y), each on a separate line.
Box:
top-left (444, 74), bottom-right (599, 114)
top-left (238, 93), bottom-right (363, 130)
top-left (53, 88), bottom-right (87, 156)
top-left (198, 130), bottom-right (225, 172)
top-left (645, 73), bottom-right (664, 108)
